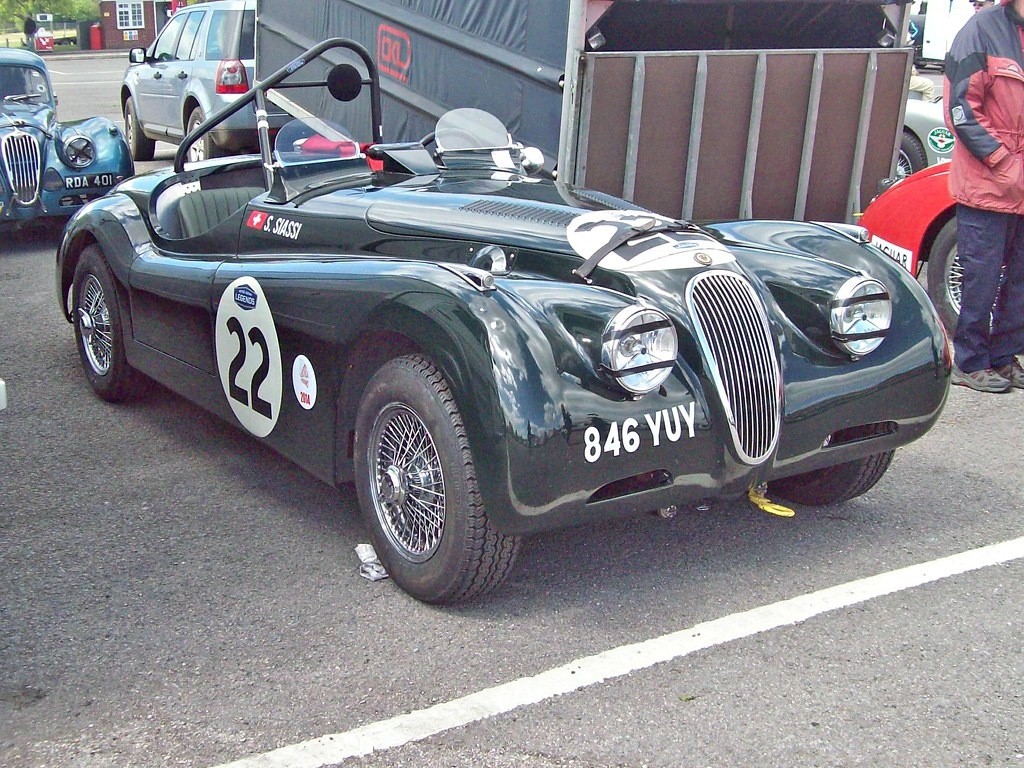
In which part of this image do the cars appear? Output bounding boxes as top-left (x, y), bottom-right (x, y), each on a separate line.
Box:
top-left (858, 162), bottom-right (1007, 344)
top-left (0, 48), bottom-right (136, 227)
top-left (896, 97), bottom-right (957, 185)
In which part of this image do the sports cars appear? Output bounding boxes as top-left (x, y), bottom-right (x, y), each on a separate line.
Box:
top-left (50, 36), bottom-right (956, 607)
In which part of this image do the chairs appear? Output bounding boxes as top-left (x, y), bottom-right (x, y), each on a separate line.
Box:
top-left (178, 186), bottom-right (266, 240)
top-left (285, 166), bottom-right (373, 203)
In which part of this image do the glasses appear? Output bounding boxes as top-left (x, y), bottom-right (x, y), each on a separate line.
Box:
top-left (973, 2), bottom-right (992, 7)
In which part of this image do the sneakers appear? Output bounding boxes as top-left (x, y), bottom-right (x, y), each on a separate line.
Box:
top-left (951, 361), bottom-right (1012, 394)
top-left (992, 355), bottom-right (1024, 389)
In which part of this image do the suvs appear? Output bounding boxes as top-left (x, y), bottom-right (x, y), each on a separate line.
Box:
top-left (117, 2), bottom-right (296, 165)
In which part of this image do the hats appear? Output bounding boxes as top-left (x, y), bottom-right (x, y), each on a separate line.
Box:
top-left (970, 1), bottom-right (996, 3)
top-left (905, 32), bottom-right (915, 46)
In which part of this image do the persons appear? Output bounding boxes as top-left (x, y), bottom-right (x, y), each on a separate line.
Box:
top-left (906, 31), bottom-right (935, 101)
top-left (970, 1), bottom-right (995, 13)
top-left (24, 14), bottom-right (36, 47)
top-left (942, 0), bottom-right (1024, 394)
